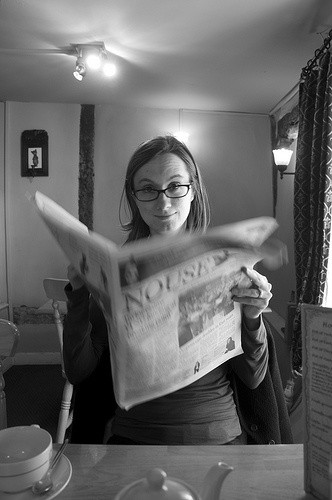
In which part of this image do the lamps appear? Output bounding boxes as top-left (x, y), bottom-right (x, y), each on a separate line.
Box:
top-left (70, 44), bottom-right (116, 81)
top-left (272, 148), bottom-right (295, 180)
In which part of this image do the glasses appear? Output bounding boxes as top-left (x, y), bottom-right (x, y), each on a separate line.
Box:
top-left (129, 182), bottom-right (191, 203)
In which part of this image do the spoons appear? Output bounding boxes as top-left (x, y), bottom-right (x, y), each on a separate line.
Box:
top-left (31, 439), bottom-right (69, 495)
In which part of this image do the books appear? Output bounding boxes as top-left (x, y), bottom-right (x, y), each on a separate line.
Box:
top-left (34, 190), bottom-right (279, 411)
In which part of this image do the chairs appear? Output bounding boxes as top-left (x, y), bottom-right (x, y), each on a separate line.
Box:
top-left (0, 278), bottom-right (67, 444)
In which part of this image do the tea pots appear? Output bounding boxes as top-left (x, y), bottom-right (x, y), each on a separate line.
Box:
top-left (113, 461), bottom-right (234, 500)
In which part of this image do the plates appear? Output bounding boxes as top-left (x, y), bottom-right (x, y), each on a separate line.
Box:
top-left (0, 450), bottom-right (73, 500)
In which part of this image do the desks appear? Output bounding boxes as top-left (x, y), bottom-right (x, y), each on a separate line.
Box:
top-left (50, 444), bottom-right (305, 500)
top-left (12, 324), bottom-right (62, 366)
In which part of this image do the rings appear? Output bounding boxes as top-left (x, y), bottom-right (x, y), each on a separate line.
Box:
top-left (257, 288), bottom-right (263, 298)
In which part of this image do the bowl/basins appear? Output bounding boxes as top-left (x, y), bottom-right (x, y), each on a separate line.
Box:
top-left (0, 424), bottom-right (54, 493)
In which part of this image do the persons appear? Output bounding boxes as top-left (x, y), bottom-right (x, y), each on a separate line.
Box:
top-left (178, 271), bottom-right (242, 347)
top-left (63, 134), bottom-right (298, 446)
top-left (225, 337), bottom-right (236, 353)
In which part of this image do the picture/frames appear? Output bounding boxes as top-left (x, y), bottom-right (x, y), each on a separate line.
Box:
top-left (21, 130), bottom-right (48, 176)
